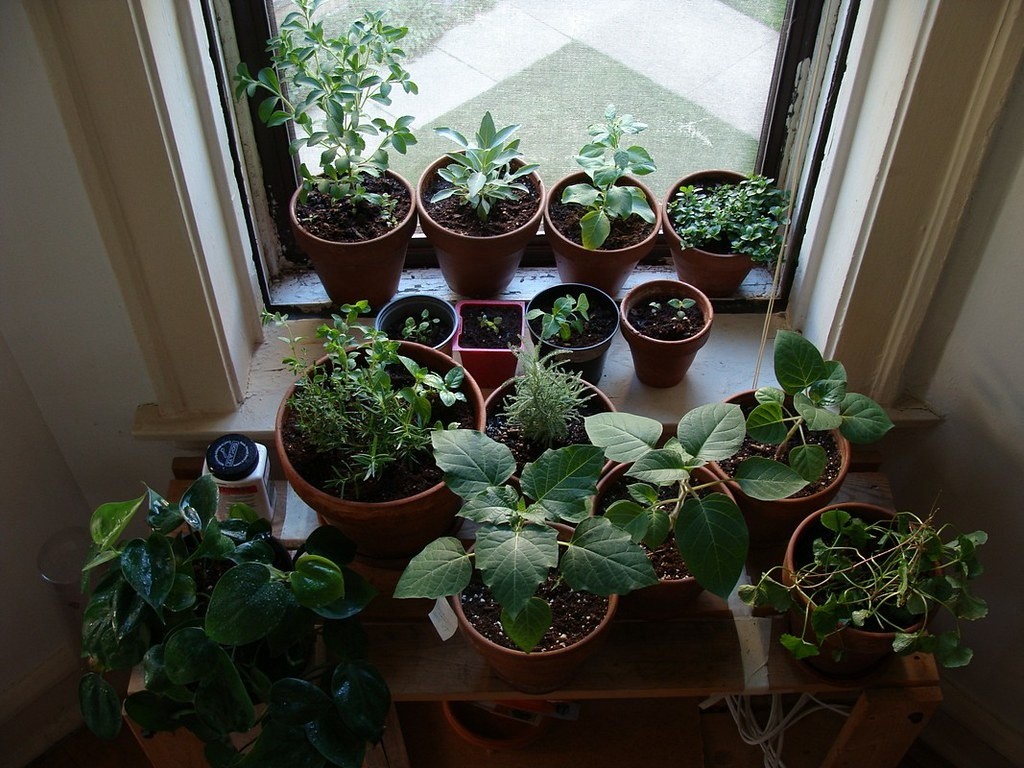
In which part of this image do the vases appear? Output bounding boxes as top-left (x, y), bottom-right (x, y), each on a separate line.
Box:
top-left (442, 700), bottom-right (553, 751)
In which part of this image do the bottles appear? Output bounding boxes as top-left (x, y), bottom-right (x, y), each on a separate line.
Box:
top-left (200, 433), bottom-right (276, 524)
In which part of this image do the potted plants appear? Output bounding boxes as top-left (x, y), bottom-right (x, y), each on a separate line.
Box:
top-left (233, 0), bottom-right (413, 320)
top-left (396, 430), bottom-right (655, 693)
top-left (527, 285), bottom-right (618, 384)
top-left (586, 401), bottom-right (747, 621)
top-left (619, 278), bottom-right (713, 387)
top-left (480, 371), bottom-right (619, 482)
top-left (450, 299), bottom-right (526, 388)
top-left (758, 502), bottom-right (943, 667)
top-left (371, 290), bottom-right (458, 359)
top-left (74, 474), bottom-right (390, 768)
top-left (415, 112), bottom-right (545, 298)
top-left (263, 301), bottom-right (486, 550)
top-left (664, 169), bottom-right (783, 298)
top-left (544, 102), bottom-right (659, 296)
top-left (700, 332), bottom-right (895, 542)
